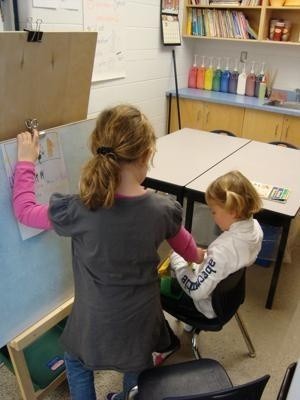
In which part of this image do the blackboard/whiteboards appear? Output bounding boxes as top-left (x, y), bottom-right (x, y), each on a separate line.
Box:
top-left (83, 0), bottom-right (127, 82)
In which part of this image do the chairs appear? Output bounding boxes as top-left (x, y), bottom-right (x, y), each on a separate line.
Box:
top-left (139, 268), bottom-right (270, 400)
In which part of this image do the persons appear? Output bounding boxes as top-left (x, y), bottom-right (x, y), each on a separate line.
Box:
top-left (149, 168), bottom-right (268, 325)
top-left (9, 103), bottom-right (206, 399)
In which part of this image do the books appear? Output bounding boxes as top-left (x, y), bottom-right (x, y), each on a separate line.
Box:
top-left (186, 0), bottom-right (264, 40)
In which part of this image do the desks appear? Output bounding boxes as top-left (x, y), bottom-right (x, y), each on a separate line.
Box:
top-left (142, 128), bottom-right (300, 309)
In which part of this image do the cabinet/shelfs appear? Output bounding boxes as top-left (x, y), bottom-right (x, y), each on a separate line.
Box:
top-left (169, 97), bottom-right (299, 149)
top-left (182, 0), bottom-right (300, 46)
